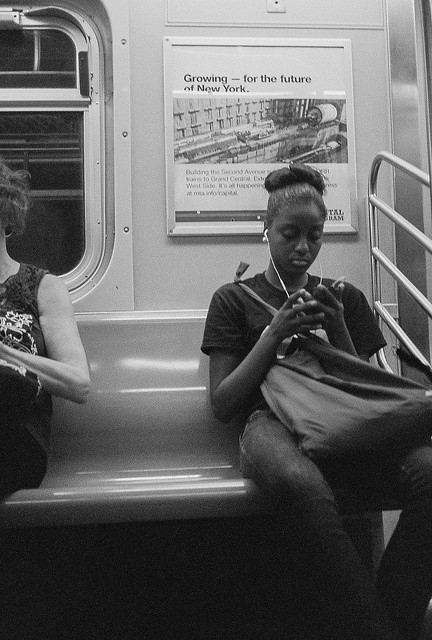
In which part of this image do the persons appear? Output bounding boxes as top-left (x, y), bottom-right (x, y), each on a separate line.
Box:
top-left (201, 162), bottom-right (432, 640)
top-left (0, 161), bottom-right (89, 505)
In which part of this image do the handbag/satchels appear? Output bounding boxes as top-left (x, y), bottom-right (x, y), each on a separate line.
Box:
top-left (233, 262), bottom-right (432, 464)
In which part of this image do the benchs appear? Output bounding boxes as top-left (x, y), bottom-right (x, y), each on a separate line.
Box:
top-left (1, 314), bottom-right (389, 524)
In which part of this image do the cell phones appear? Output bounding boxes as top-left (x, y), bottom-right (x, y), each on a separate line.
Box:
top-left (316, 285), bottom-right (343, 307)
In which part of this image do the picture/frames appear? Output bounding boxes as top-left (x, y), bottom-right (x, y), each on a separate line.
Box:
top-left (162, 36), bottom-right (361, 238)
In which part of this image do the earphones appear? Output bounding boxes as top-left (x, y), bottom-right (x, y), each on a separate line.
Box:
top-left (263, 228), bottom-right (323, 298)
